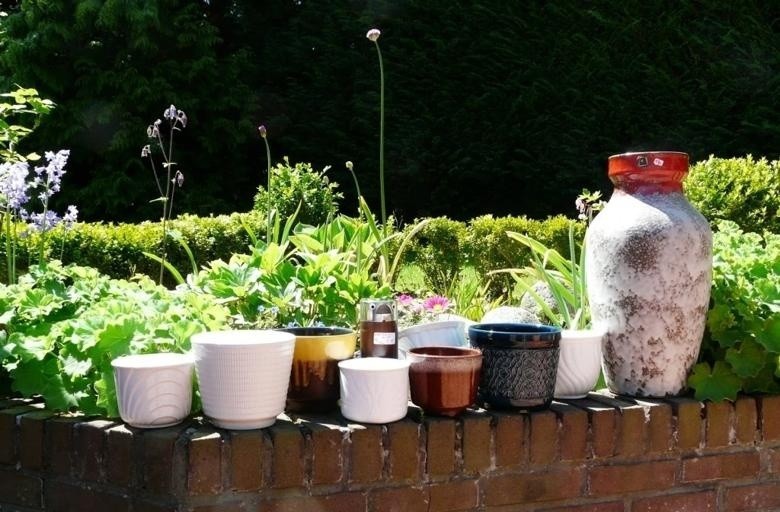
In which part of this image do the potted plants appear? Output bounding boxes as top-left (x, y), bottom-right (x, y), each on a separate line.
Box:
top-left (485, 218), bottom-right (604, 401)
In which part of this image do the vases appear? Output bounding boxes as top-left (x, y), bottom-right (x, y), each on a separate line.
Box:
top-left (397, 313), bottom-right (469, 402)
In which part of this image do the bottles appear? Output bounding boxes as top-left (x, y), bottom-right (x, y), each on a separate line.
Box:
top-left (361, 300), bottom-right (398, 358)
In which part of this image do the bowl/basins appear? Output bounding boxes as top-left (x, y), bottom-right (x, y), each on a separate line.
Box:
top-left (553, 327), bottom-right (605, 399)
top-left (396, 320), bottom-right (468, 354)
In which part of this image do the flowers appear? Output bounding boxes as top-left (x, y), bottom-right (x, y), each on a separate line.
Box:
top-left (396, 296), bottom-right (447, 326)
